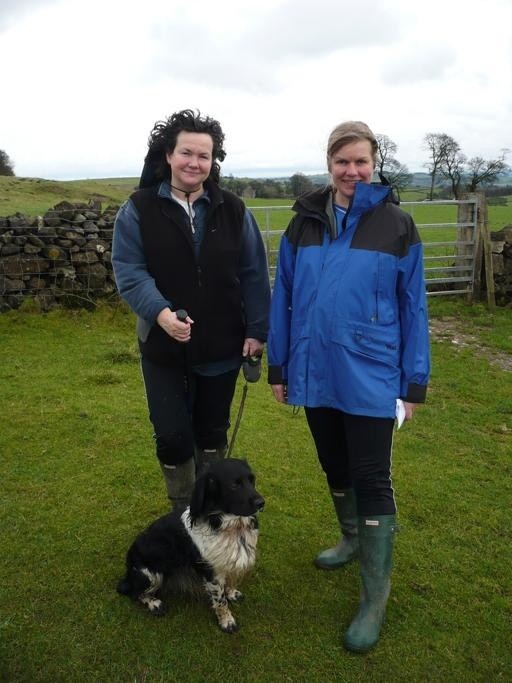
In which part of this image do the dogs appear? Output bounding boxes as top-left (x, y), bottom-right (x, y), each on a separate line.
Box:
top-left (116, 456), bottom-right (266, 634)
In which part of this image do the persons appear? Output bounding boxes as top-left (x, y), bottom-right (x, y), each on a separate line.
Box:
top-left (112, 111), bottom-right (269, 516)
top-left (264, 120), bottom-right (432, 653)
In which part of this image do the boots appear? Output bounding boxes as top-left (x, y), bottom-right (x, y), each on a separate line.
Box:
top-left (159, 459), bottom-right (196, 510)
top-left (315, 489), bottom-right (361, 570)
top-left (193, 444), bottom-right (229, 482)
top-left (343, 515), bottom-right (394, 651)
